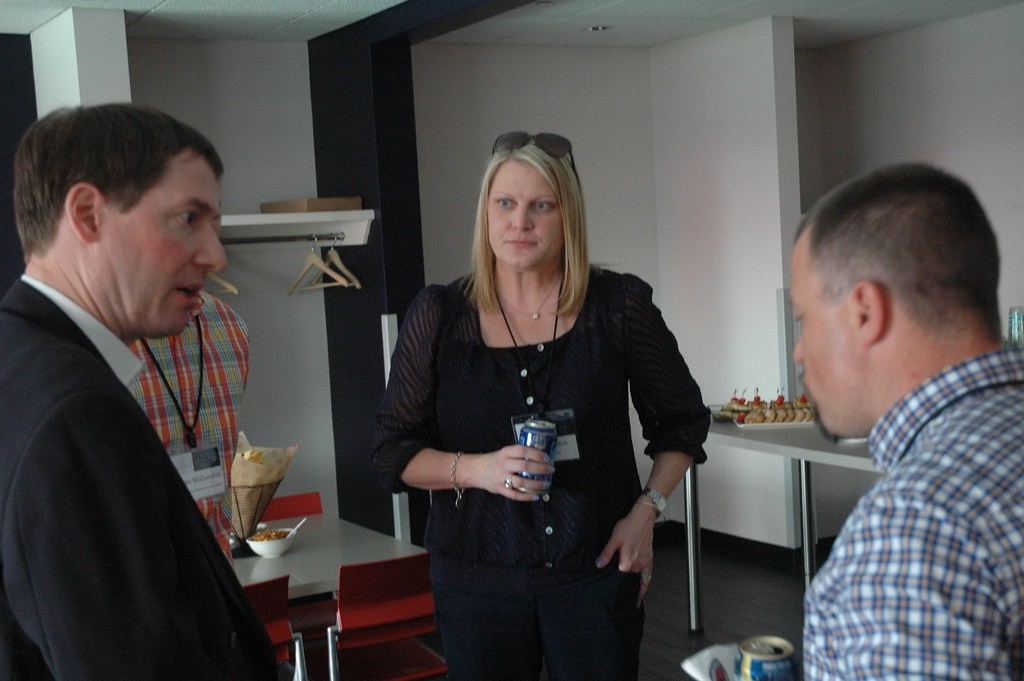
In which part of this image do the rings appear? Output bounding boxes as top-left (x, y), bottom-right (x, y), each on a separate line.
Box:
top-left (504, 473), bottom-right (513, 490)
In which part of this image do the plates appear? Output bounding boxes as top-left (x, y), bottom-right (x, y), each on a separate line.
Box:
top-left (732, 417), bottom-right (817, 431)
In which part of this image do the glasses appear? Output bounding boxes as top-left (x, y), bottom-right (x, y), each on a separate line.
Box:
top-left (491, 132), bottom-right (581, 192)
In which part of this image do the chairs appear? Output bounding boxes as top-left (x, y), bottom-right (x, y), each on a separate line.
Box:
top-left (287, 551), bottom-right (449, 681)
top-left (258, 491), bottom-right (323, 521)
top-left (238, 575), bottom-right (307, 681)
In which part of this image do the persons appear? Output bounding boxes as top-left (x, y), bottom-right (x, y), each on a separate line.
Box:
top-left (370, 131), bottom-right (711, 681)
top-left (125, 289), bottom-right (250, 577)
top-left (0, 105), bottom-right (279, 681)
top-left (790, 161), bottom-right (1024, 681)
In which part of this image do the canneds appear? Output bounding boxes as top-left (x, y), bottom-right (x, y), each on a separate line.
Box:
top-left (515, 420), bottom-right (557, 495)
top-left (733, 634), bottom-right (800, 681)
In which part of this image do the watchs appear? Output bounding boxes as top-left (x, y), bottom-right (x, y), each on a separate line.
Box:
top-left (641, 487), bottom-right (667, 517)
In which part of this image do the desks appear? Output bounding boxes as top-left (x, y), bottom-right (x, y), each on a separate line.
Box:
top-left (681, 413), bottom-right (884, 637)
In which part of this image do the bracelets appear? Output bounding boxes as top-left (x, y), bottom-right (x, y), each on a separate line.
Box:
top-left (635, 501), bottom-right (666, 521)
top-left (450, 450), bottom-right (466, 509)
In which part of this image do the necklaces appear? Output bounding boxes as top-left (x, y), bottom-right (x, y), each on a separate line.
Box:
top-left (498, 275), bottom-right (560, 319)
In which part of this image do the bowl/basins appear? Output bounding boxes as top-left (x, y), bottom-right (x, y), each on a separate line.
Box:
top-left (246, 528), bottom-right (297, 559)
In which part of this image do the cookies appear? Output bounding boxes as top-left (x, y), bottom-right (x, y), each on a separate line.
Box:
top-left (744, 407), bottom-right (815, 424)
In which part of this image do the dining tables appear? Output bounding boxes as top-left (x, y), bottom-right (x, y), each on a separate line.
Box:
top-left (228, 513), bottom-right (429, 600)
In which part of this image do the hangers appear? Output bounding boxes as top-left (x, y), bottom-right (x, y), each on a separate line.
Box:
top-left (299, 237), bottom-right (361, 289)
top-left (286, 237), bottom-right (350, 296)
top-left (202, 272), bottom-right (239, 295)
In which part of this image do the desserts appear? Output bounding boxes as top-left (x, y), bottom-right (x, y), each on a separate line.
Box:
top-left (720, 389), bottom-right (812, 411)
